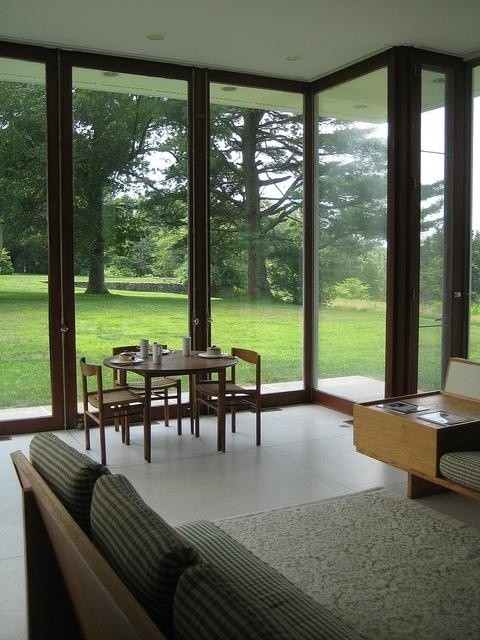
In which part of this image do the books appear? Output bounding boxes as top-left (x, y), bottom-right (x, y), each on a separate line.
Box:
top-left (417, 408), bottom-right (475, 427)
top-left (375, 399), bottom-right (430, 415)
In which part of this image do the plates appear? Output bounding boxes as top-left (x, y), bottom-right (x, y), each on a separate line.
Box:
top-left (148, 348), bottom-right (170, 355)
top-left (198, 352), bottom-right (229, 359)
top-left (110, 357), bottom-right (144, 366)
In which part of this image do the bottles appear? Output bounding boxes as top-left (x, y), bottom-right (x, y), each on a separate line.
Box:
top-left (182, 337), bottom-right (191, 357)
top-left (140, 339), bottom-right (149, 359)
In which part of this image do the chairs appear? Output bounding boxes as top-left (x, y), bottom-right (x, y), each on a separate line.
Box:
top-left (79, 344), bottom-right (262, 464)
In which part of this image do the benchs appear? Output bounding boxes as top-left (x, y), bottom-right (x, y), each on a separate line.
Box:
top-left (11, 449), bottom-right (377, 640)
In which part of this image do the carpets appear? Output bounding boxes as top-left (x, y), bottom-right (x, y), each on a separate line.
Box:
top-left (218, 486), bottom-right (480, 638)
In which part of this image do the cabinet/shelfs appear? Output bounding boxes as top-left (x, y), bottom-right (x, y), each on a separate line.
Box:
top-left (353, 358), bottom-right (480, 503)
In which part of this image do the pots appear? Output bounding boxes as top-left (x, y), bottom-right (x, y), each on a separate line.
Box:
top-left (153, 342), bottom-right (163, 365)
